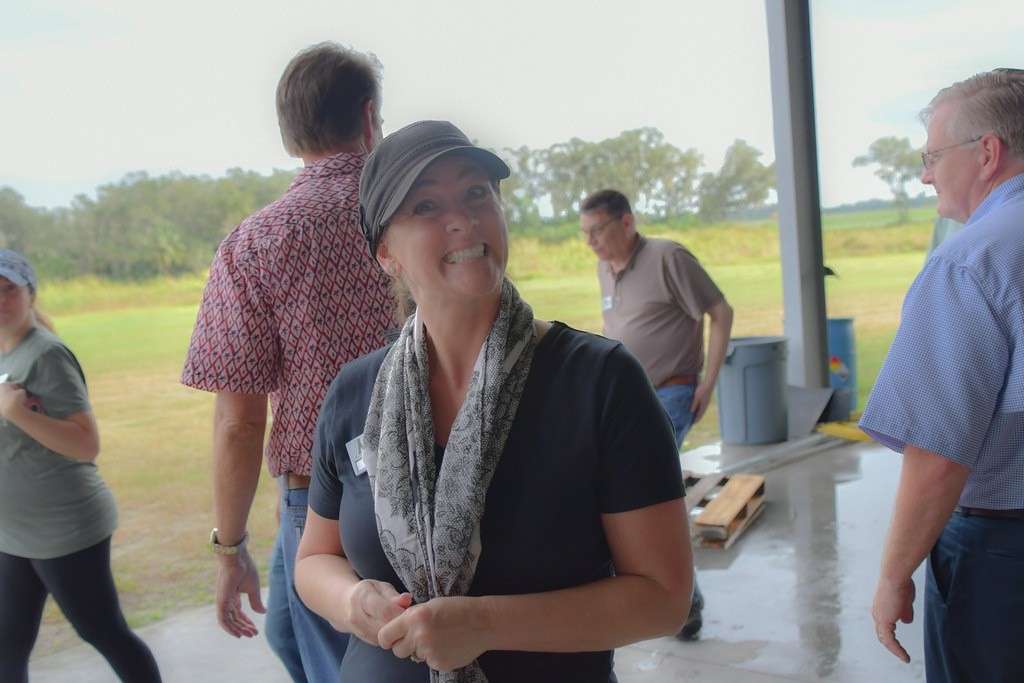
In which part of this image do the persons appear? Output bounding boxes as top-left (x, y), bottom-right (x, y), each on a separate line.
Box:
top-left (578, 188), bottom-right (735, 642)
top-left (295, 121), bottom-right (697, 683)
top-left (0, 246), bottom-right (160, 683)
top-left (855, 67), bottom-right (1024, 683)
top-left (180, 41), bottom-right (416, 683)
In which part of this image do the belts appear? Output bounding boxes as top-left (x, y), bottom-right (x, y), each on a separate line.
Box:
top-left (663, 374), bottom-right (700, 386)
top-left (286, 471), bottom-right (311, 490)
top-left (955, 505), bottom-right (1023, 518)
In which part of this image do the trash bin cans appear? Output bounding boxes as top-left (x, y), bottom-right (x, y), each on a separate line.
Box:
top-left (827, 318), bottom-right (858, 421)
top-left (717, 334), bottom-right (790, 446)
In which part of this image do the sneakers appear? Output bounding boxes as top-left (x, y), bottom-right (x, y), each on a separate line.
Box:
top-left (673, 593), bottom-right (704, 640)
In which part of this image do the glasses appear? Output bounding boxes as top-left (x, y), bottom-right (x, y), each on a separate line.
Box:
top-left (921, 135), bottom-right (1008, 171)
top-left (577, 218), bottom-right (625, 238)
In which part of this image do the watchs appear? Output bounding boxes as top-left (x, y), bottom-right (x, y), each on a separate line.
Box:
top-left (208, 528), bottom-right (250, 555)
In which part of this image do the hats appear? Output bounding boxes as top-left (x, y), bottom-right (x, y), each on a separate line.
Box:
top-left (0, 248), bottom-right (37, 291)
top-left (358, 119), bottom-right (510, 240)
